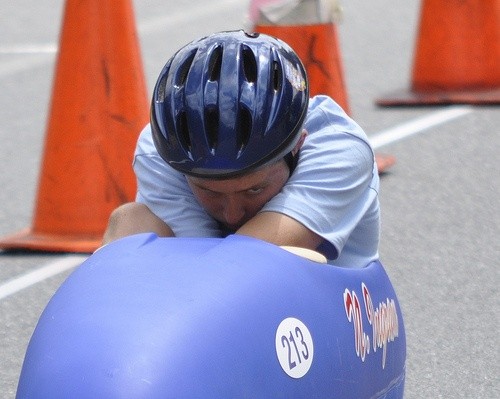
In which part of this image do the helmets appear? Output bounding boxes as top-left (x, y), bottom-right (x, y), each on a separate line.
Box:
top-left (150, 29), bottom-right (308, 180)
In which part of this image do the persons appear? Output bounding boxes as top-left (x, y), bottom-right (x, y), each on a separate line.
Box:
top-left (96, 28), bottom-right (382, 271)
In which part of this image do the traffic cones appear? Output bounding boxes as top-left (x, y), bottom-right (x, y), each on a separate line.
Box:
top-left (371, 0), bottom-right (500, 112)
top-left (248, 1), bottom-right (397, 176)
top-left (1, 0), bottom-right (161, 252)
